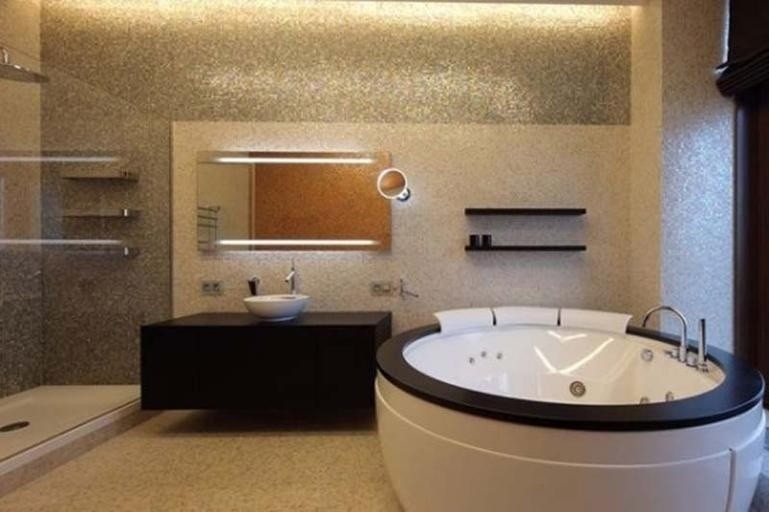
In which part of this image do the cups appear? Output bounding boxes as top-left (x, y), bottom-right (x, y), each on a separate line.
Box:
top-left (469, 234), bottom-right (480, 245)
top-left (481, 235), bottom-right (493, 247)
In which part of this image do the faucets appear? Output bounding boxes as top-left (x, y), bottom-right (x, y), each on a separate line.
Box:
top-left (285, 256), bottom-right (296, 294)
top-left (640, 305), bottom-right (689, 363)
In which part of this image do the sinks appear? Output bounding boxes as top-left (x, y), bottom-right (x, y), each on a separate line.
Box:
top-left (243, 294), bottom-right (311, 321)
top-left (402, 322), bottom-right (727, 407)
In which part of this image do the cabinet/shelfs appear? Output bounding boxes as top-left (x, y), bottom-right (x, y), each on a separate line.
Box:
top-left (195, 201), bottom-right (221, 251)
top-left (463, 207), bottom-right (587, 253)
top-left (58, 172), bottom-right (140, 258)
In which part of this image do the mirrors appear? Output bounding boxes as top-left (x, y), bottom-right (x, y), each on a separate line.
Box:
top-left (373, 167), bottom-right (406, 200)
top-left (190, 147), bottom-right (391, 256)
